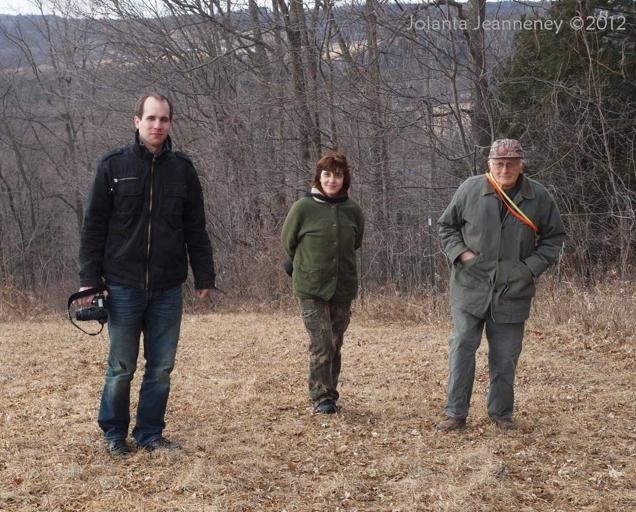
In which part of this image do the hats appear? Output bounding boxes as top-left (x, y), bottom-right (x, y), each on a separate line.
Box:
top-left (488, 138), bottom-right (524, 161)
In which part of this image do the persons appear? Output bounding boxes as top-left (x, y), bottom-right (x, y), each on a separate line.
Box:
top-left (71, 92), bottom-right (216, 458)
top-left (281, 153), bottom-right (364, 414)
top-left (436, 138), bottom-right (565, 431)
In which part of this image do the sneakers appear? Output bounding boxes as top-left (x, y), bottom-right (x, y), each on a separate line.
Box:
top-left (109, 440), bottom-right (129, 455)
top-left (435, 415), bottom-right (466, 433)
top-left (495, 420), bottom-right (514, 429)
top-left (144, 437), bottom-right (180, 449)
top-left (314, 400), bottom-right (337, 414)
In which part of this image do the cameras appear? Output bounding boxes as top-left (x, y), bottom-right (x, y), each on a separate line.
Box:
top-left (75, 293), bottom-right (108, 324)
